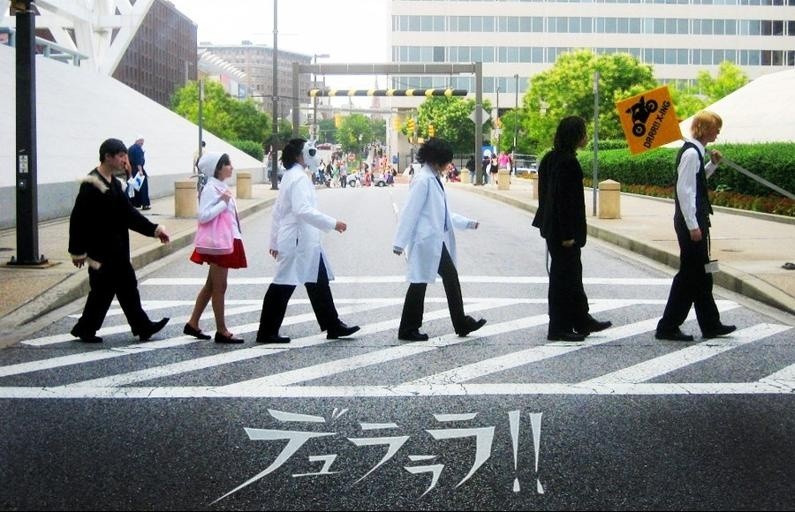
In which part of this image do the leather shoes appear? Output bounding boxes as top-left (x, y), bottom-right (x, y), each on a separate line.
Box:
top-left (575, 313), bottom-right (612, 337)
top-left (140, 318), bottom-right (170, 341)
top-left (547, 331), bottom-right (584, 341)
top-left (215, 332), bottom-right (244, 344)
top-left (70, 323), bottom-right (103, 343)
top-left (327, 318), bottom-right (360, 339)
top-left (702, 322), bottom-right (736, 339)
top-left (183, 323), bottom-right (211, 340)
top-left (656, 327), bottom-right (694, 341)
top-left (256, 335), bottom-right (290, 343)
top-left (459, 315), bottom-right (487, 337)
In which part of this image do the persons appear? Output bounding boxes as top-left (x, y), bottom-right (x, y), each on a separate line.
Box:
top-left (653, 108), bottom-right (737, 342)
top-left (66, 137), bottom-right (173, 344)
top-left (112, 149), bottom-right (133, 197)
top-left (191, 140), bottom-right (209, 175)
top-left (390, 134), bottom-right (488, 342)
top-left (441, 150), bottom-right (514, 187)
top-left (127, 137), bottom-right (152, 210)
top-left (315, 141), bottom-right (398, 189)
top-left (255, 137), bottom-right (360, 344)
top-left (180, 149), bottom-right (248, 344)
top-left (529, 113), bottom-right (614, 342)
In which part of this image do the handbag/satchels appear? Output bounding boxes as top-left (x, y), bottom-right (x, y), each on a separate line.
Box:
top-left (193, 209), bottom-right (234, 255)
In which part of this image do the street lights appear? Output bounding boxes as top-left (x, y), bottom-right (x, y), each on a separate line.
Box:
top-left (494, 84), bottom-right (503, 155)
top-left (311, 53), bottom-right (331, 140)
top-left (590, 69), bottom-right (605, 218)
top-left (510, 73), bottom-right (522, 178)
top-left (196, 78), bottom-right (209, 160)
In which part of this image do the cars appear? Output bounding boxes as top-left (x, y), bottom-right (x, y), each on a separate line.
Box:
top-left (509, 166), bottom-right (540, 180)
top-left (345, 169), bottom-right (393, 188)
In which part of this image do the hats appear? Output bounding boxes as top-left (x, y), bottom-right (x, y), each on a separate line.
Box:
top-left (198, 152), bottom-right (225, 177)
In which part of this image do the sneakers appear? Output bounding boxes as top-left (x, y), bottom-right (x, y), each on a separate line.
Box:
top-left (142, 206), bottom-right (151, 209)
top-left (399, 330), bottom-right (428, 340)
